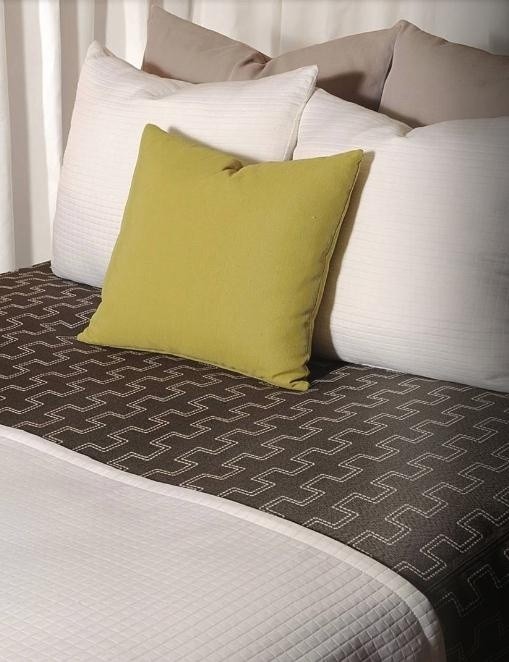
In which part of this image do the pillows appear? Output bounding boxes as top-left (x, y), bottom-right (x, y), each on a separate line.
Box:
top-left (49, 39), bottom-right (320, 289)
top-left (291, 90), bottom-right (412, 163)
top-left (137, 0), bottom-right (403, 112)
top-left (296, 83), bottom-right (508, 396)
top-left (73, 124), bottom-right (363, 392)
top-left (373, 15), bottom-right (508, 125)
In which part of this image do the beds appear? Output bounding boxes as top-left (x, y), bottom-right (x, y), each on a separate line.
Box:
top-left (1, 1), bottom-right (509, 659)
